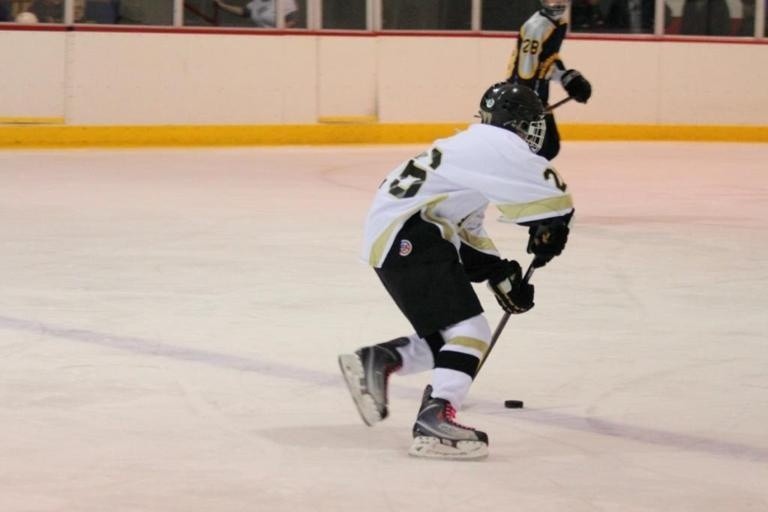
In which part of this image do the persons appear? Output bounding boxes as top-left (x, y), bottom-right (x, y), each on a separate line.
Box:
top-left (349, 80), bottom-right (576, 448)
top-left (503, 1), bottom-right (593, 166)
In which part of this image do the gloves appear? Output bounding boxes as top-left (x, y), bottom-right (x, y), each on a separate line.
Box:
top-left (560, 69), bottom-right (591, 103)
top-left (526, 213), bottom-right (572, 268)
top-left (486, 260), bottom-right (535, 314)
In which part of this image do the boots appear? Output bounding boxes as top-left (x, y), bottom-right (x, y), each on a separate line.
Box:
top-left (413, 384), bottom-right (489, 446)
top-left (356, 336), bottom-right (410, 418)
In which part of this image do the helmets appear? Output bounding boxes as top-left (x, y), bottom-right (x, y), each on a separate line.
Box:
top-left (479, 82), bottom-right (549, 116)
top-left (541, 0), bottom-right (567, 17)
